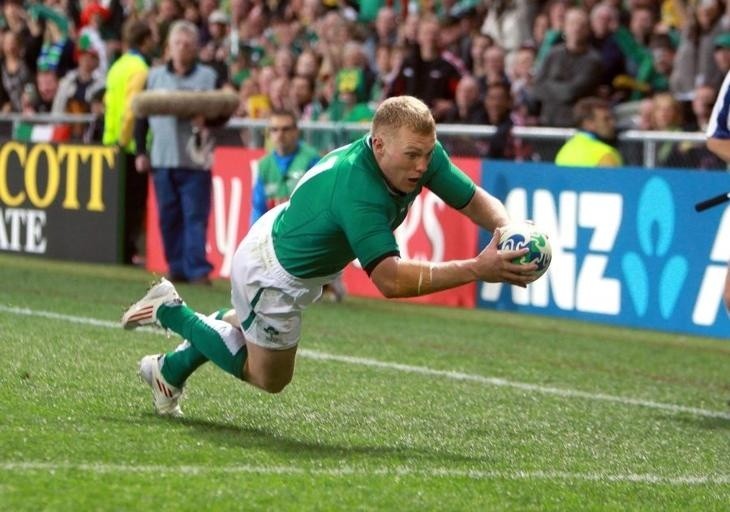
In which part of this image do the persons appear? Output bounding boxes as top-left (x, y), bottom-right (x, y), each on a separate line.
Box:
top-left (704, 71), bottom-right (730, 314)
top-left (131, 19), bottom-right (242, 290)
top-left (98, 22), bottom-right (159, 267)
top-left (1, 0), bottom-right (729, 172)
top-left (249, 108), bottom-right (345, 305)
top-left (118, 95), bottom-right (552, 424)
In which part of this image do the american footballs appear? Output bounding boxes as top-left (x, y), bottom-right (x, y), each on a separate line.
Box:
top-left (496, 221), bottom-right (553, 284)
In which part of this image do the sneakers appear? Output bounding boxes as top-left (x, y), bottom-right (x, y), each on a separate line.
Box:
top-left (167, 276), bottom-right (215, 285)
top-left (119, 276), bottom-right (188, 331)
top-left (324, 276), bottom-right (343, 304)
top-left (137, 352), bottom-right (184, 419)
